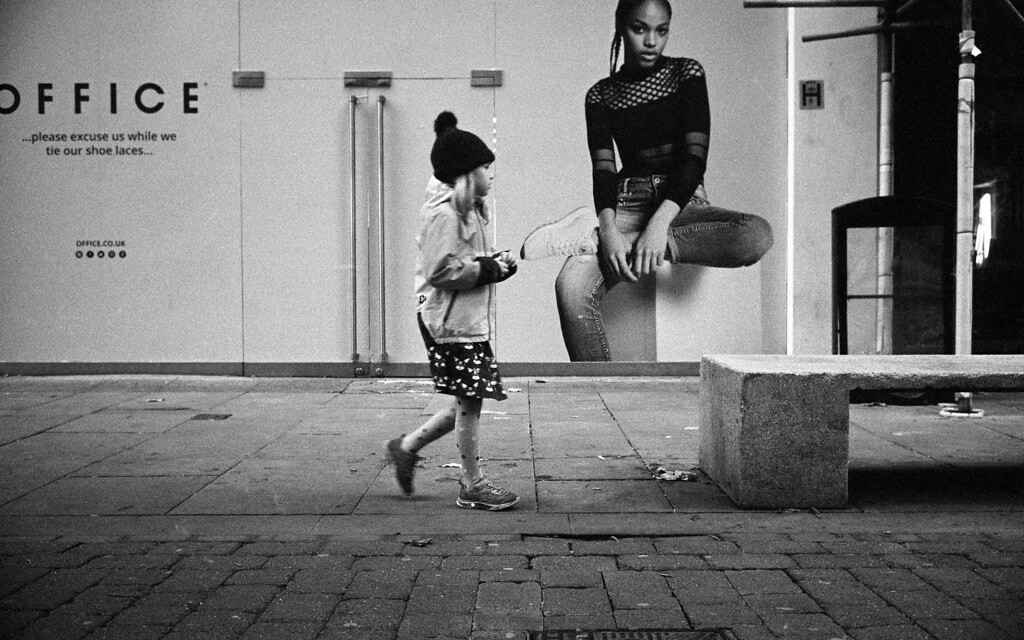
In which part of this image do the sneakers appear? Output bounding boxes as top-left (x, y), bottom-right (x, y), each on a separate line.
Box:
top-left (520, 207), bottom-right (599, 261)
top-left (386, 432), bottom-right (423, 497)
top-left (455, 475), bottom-right (522, 512)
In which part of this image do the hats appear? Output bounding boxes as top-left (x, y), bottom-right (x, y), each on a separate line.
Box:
top-left (430, 111), bottom-right (495, 183)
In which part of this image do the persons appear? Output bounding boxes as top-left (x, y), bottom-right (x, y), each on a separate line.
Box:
top-left (381, 111), bottom-right (519, 512)
top-left (521, 0), bottom-right (771, 362)
